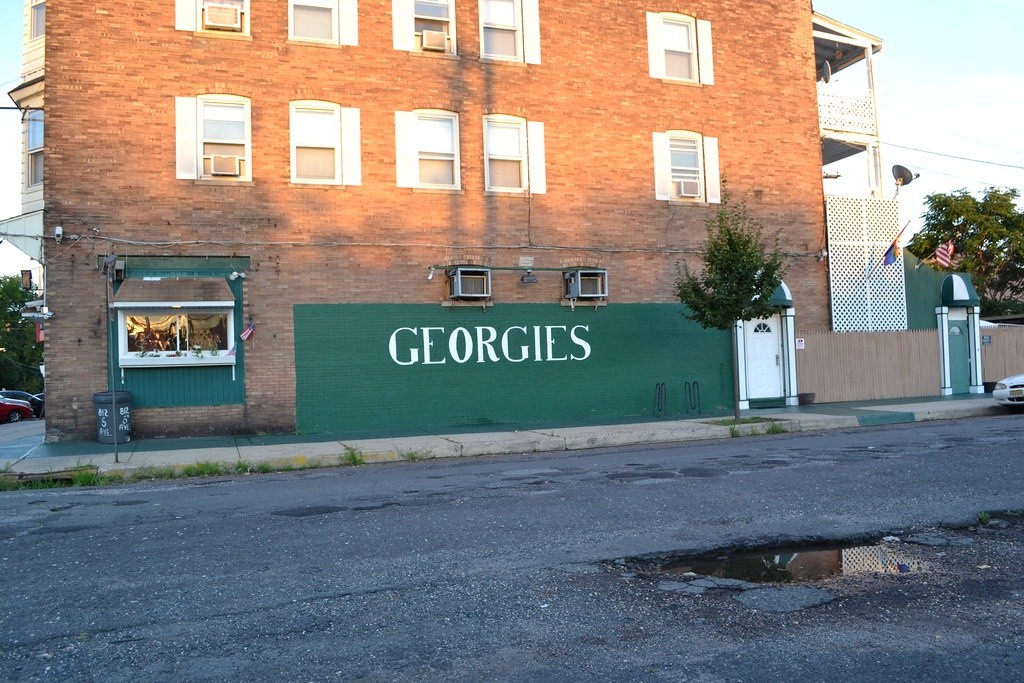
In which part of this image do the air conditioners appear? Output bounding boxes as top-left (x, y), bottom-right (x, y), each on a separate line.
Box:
top-left (420, 31), bottom-right (447, 51)
top-left (204, 2), bottom-right (242, 31)
top-left (211, 155), bottom-right (240, 175)
top-left (568, 277), bottom-right (601, 295)
top-left (675, 181), bottom-right (700, 197)
top-left (450, 275), bottom-right (487, 295)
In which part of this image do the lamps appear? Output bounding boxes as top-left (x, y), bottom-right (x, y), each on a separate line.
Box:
top-left (833, 41), bottom-right (843, 59)
top-left (521, 270), bottom-right (537, 283)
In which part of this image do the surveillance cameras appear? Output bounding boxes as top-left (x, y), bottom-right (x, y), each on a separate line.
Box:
top-left (55, 227), bottom-right (63, 240)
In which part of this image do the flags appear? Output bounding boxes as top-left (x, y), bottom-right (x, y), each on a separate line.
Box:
top-left (934, 238), bottom-right (954, 269)
top-left (883, 224), bottom-right (908, 267)
top-left (239, 321), bottom-right (257, 341)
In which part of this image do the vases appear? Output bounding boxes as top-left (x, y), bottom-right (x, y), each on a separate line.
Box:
top-left (797, 393), bottom-right (816, 404)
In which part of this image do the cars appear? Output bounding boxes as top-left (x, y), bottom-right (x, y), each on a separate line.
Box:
top-left (0, 391), bottom-right (45, 424)
top-left (993, 374), bottom-right (1024, 414)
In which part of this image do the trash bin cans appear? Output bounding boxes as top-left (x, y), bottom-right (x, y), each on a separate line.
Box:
top-left (92, 389), bottom-right (133, 444)
